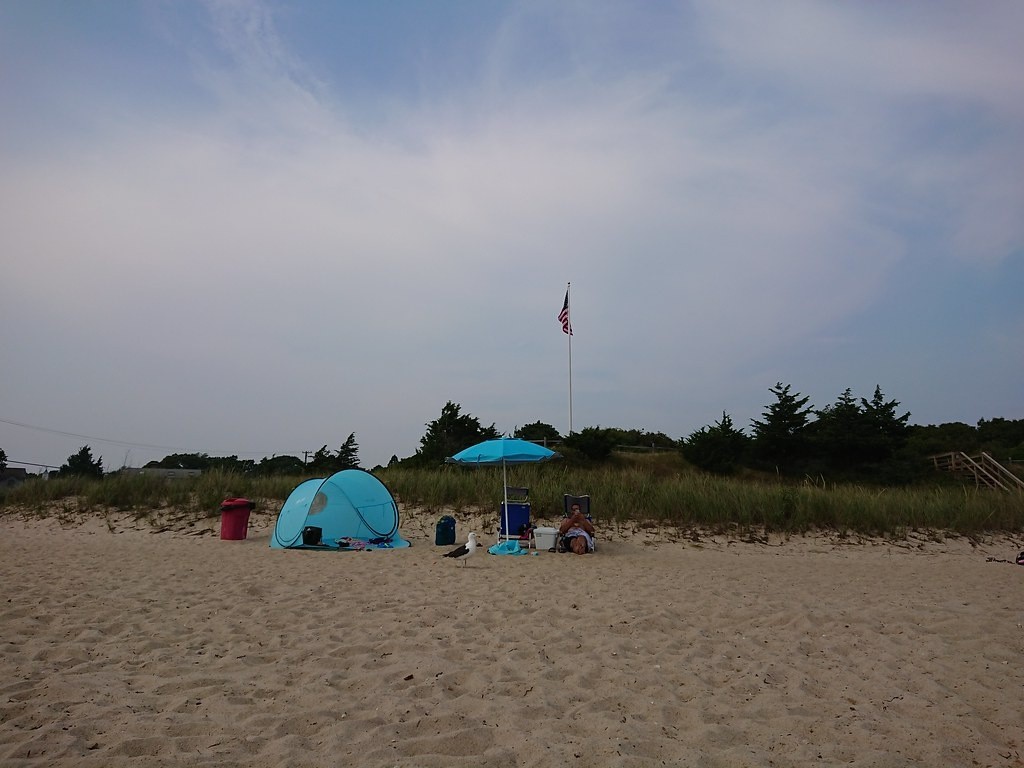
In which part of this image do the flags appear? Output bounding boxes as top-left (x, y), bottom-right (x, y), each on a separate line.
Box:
top-left (556, 287), bottom-right (574, 336)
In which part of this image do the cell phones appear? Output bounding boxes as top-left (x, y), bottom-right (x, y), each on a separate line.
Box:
top-left (575, 509), bottom-right (579, 515)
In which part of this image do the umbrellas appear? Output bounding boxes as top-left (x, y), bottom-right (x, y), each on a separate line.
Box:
top-left (443, 436), bottom-right (556, 540)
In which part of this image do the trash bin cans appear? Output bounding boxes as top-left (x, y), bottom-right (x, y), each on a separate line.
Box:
top-left (220, 498), bottom-right (251, 540)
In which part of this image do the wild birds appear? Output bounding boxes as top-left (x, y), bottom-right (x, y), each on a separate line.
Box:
top-left (439, 532), bottom-right (481, 568)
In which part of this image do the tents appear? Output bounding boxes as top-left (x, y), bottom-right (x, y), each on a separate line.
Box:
top-left (269, 467), bottom-right (412, 551)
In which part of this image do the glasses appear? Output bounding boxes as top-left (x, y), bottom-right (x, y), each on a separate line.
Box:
top-left (571, 508), bottom-right (580, 512)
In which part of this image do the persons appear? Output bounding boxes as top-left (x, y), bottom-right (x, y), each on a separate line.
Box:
top-left (558, 503), bottom-right (595, 555)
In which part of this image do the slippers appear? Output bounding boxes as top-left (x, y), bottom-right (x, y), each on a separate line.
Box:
top-left (559, 547), bottom-right (566, 552)
top-left (548, 547), bottom-right (556, 553)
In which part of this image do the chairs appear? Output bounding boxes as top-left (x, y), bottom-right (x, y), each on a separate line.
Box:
top-left (497, 486), bottom-right (536, 549)
top-left (557, 493), bottom-right (597, 553)
